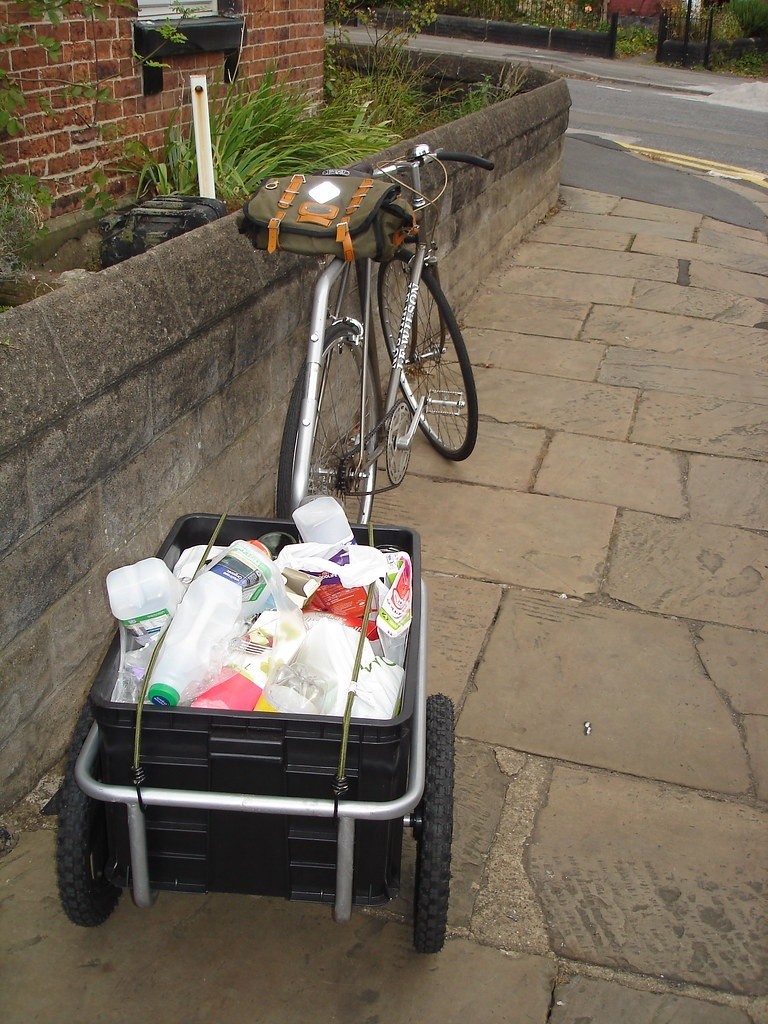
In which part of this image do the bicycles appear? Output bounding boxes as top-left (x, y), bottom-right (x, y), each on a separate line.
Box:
top-left (272, 141), bottom-right (494, 520)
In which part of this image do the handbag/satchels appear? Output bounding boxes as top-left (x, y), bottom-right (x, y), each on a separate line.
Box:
top-left (241, 173), bottom-right (419, 265)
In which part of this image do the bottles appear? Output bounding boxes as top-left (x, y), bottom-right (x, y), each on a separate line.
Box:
top-left (193, 540), bottom-right (273, 620)
top-left (292, 497), bottom-right (357, 566)
top-left (105, 558), bottom-right (186, 646)
top-left (147, 569), bottom-right (242, 706)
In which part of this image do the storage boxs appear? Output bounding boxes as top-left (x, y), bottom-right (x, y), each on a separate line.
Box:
top-left (90, 512), bottom-right (422, 906)
